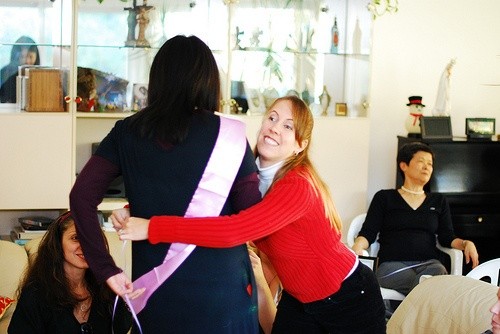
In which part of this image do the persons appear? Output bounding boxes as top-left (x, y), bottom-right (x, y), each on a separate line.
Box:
top-left (482, 287), bottom-right (500, 334)
top-left (247, 240), bottom-right (284, 334)
top-left (6, 210), bottom-right (133, 334)
top-left (109, 95), bottom-right (387, 334)
top-left (139, 87), bottom-right (148, 108)
top-left (70, 35), bottom-right (262, 334)
top-left (351, 142), bottom-right (479, 298)
top-left (0, 35), bottom-right (40, 104)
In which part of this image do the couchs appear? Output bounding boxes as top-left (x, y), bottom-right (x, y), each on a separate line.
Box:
top-left (0, 231), bottom-right (133, 334)
top-left (386, 276), bottom-right (500, 334)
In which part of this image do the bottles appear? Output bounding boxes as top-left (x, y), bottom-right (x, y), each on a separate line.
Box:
top-left (330, 16), bottom-right (339, 54)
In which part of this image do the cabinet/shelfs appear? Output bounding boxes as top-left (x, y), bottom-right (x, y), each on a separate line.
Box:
top-left (0, 0), bottom-right (375, 248)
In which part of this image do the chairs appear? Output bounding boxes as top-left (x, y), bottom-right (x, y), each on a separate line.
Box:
top-left (345, 213), bottom-right (500, 301)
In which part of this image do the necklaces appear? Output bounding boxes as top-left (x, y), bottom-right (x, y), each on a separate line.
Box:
top-left (81, 304), bottom-right (90, 312)
top-left (401, 186), bottom-right (424, 194)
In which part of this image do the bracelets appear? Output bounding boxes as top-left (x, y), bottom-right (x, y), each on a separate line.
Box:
top-left (463, 240), bottom-right (466, 244)
